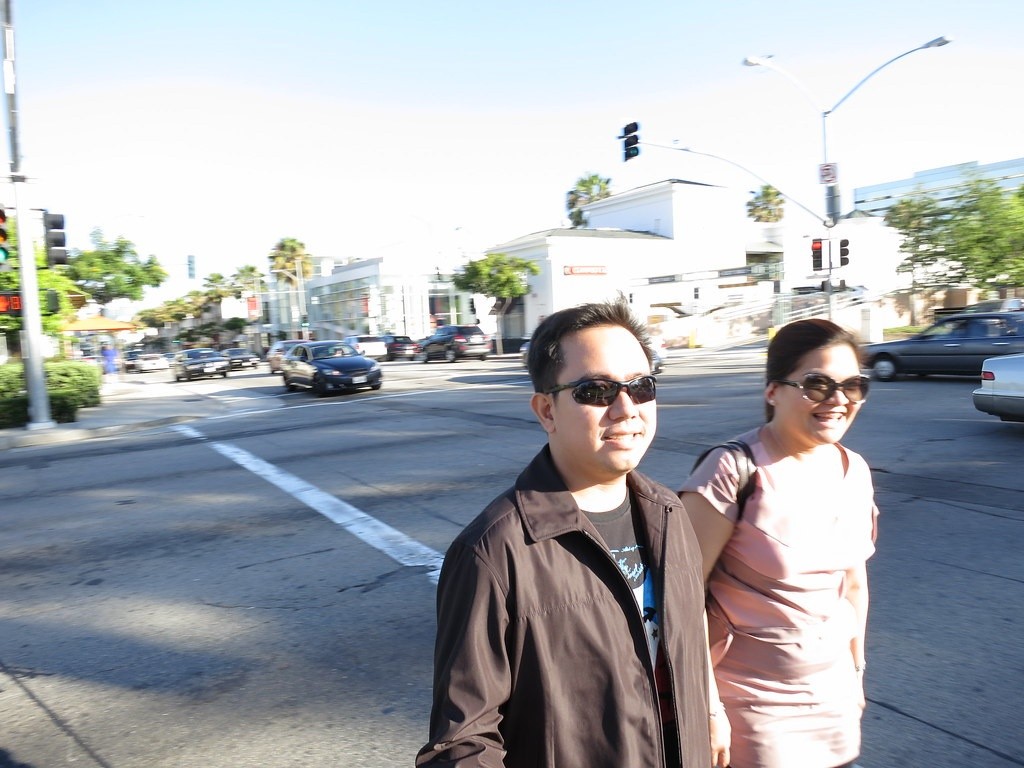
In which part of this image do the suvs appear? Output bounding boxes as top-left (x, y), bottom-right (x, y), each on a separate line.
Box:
top-left (342, 335), bottom-right (388, 360)
top-left (418, 324), bottom-right (494, 365)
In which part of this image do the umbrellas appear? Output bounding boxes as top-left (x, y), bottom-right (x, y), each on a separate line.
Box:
top-left (59, 316), bottom-right (139, 356)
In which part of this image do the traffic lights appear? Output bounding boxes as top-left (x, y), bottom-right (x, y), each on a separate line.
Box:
top-left (44, 291), bottom-right (61, 315)
top-left (812, 238), bottom-right (822, 271)
top-left (38, 213), bottom-right (67, 271)
top-left (839, 239), bottom-right (850, 266)
top-left (616, 121), bottom-right (640, 161)
top-left (0, 203), bottom-right (9, 266)
top-left (0, 288), bottom-right (22, 317)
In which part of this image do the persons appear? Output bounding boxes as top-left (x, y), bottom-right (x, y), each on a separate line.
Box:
top-left (677, 320), bottom-right (881, 768)
top-left (414, 304), bottom-right (712, 768)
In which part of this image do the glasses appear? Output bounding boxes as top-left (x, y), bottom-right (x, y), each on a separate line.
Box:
top-left (768, 372), bottom-right (873, 404)
top-left (541, 376), bottom-right (658, 407)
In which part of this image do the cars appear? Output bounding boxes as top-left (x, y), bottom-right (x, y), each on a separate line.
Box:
top-left (859, 311), bottom-right (1024, 383)
top-left (169, 348), bottom-right (232, 382)
top-left (280, 339), bottom-right (384, 397)
top-left (382, 335), bottom-right (421, 361)
top-left (120, 348), bottom-right (178, 372)
top-left (971, 351), bottom-right (1024, 422)
top-left (958, 298), bottom-right (1024, 314)
top-left (221, 348), bottom-right (262, 368)
top-left (267, 340), bottom-right (316, 374)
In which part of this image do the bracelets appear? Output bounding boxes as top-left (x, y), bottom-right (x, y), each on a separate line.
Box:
top-left (710, 702), bottom-right (726, 717)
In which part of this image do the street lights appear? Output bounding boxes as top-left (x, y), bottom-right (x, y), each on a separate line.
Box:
top-left (231, 282), bottom-right (265, 317)
top-left (269, 269), bottom-right (311, 340)
top-left (739, 34), bottom-right (954, 335)
